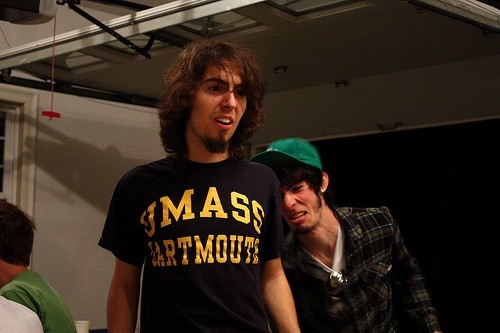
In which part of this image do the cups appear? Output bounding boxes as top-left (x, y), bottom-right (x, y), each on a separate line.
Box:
top-left (74, 321), bottom-right (91, 333)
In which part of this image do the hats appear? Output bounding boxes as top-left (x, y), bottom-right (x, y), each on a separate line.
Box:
top-left (249, 138), bottom-right (323, 169)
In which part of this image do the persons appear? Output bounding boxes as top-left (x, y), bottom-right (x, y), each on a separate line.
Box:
top-left (0, 197), bottom-right (77, 333)
top-left (248, 137), bottom-right (440, 333)
top-left (97, 37), bottom-right (303, 333)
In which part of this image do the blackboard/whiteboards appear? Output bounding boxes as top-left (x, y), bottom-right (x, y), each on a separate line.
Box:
top-left (251, 116), bottom-right (500, 333)
